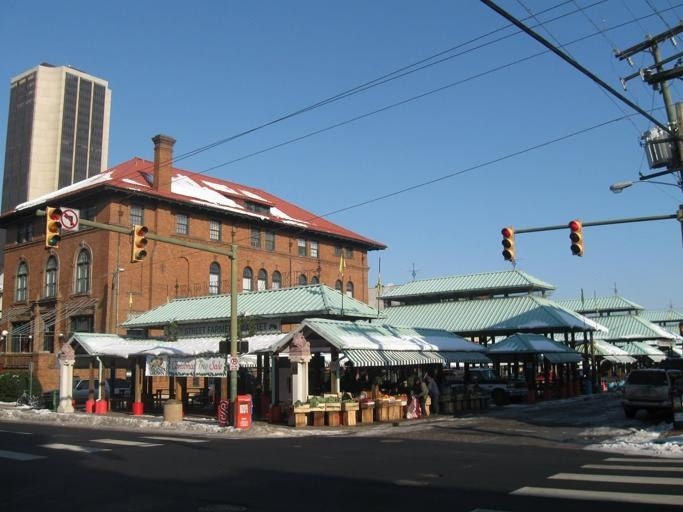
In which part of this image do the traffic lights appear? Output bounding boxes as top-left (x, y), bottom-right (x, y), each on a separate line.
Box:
top-left (568, 220), bottom-right (584, 257)
top-left (501, 227), bottom-right (516, 261)
top-left (131, 224), bottom-right (148, 263)
top-left (45, 206), bottom-right (63, 249)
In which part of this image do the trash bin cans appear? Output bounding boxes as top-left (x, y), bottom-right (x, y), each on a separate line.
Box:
top-left (163, 399), bottom-right (183, 421)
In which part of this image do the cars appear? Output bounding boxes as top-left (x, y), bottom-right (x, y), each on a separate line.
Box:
top-left (621, 367), bottom-right (671, 420)
top-left (73, 378), bottom-right (131, 404)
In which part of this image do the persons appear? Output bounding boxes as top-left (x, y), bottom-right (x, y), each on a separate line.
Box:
top-left (397, 376), bottom-right (441, 415)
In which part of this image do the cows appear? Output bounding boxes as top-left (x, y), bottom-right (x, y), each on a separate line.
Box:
top-left (292, 330), bottom-right (307, 349)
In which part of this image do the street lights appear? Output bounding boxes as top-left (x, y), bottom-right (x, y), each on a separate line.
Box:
top-left (609, 180), bottom-right (683, 194)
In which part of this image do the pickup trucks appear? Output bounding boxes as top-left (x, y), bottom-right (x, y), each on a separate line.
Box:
top-left (448, 368), bottom-right (528, 406)
